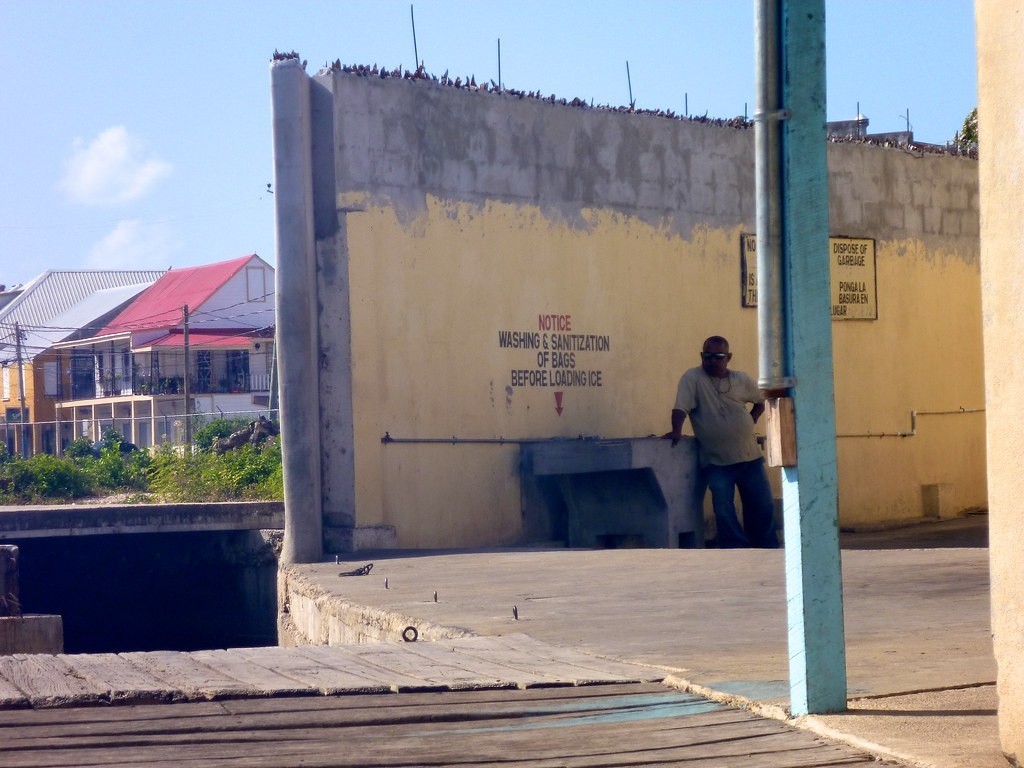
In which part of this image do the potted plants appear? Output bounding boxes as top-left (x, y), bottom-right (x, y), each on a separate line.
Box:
top-left (113, 373), bottom-right (122, 395)
top-left (176, 384), bottom-right (184, 394)
top-left (219, 379), bottom-right (240, 393)
top-left (160, 379), bottom-right (172, 395)
top-left (99, 374), bottom-right (111, 396)
top-left (138, 382), bottom-right (149, 395)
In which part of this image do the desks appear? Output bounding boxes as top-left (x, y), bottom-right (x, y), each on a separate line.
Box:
top-left (529, 435), bottom-right (708, 549)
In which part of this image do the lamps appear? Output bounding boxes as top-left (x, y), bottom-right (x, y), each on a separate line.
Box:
top-left (255, 343), bottom-right (260, 351)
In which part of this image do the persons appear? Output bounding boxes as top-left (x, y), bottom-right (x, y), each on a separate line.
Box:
top-left (662, 336), bottom-right (781, 549)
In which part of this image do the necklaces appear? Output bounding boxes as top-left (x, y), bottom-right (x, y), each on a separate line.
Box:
top-left (709, 373), bottom-right (731, 393)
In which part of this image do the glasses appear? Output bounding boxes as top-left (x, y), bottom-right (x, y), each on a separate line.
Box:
top-left (700, 352), bottom-right (727, 361)
